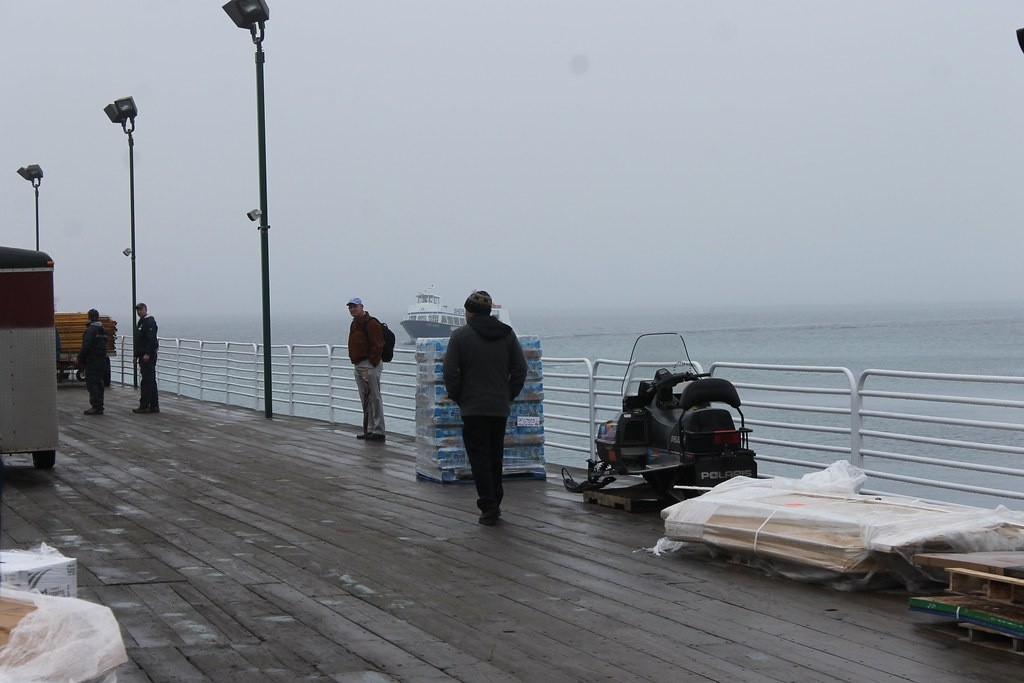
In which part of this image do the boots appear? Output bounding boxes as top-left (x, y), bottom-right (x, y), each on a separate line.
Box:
top-left (133, 379), bottom-right (150, 414)
top-left (144, 379), bottom-right (160, 413)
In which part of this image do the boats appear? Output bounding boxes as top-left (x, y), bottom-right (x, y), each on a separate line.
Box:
top-left (399, 284), bottom-right (513, 342)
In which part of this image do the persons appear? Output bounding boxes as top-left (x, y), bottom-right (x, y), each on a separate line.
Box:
top-left (132, 303), bottom-right (160, 414)
top-left (75, 309), bottom-right (106, 415)
top-left (443, 290), bottom-right (529, 526)
top-left (347, 298), bottom-right (386, 440)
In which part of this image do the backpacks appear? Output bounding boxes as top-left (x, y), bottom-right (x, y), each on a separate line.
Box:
top-left (363, 316), bottom-right (395, 363)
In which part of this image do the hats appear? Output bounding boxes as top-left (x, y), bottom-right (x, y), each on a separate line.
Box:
top-left (463, 290), bottom-right (492, 315)
top-left (135, 303), bottom-right (147, 309)
top-left (346, 297), bottom-right (363, 306)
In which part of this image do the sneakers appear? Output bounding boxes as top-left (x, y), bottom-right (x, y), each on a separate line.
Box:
top-left (366, 433), bottom-right (385, 442)
top-left (357, 433), bottom-right (373, 439)
top-left (84, 406), bottom-right (104, 414)
top-left (477, 498), bottom-right (502, 516)
top-left (478, 511), bottom-right (498, 526)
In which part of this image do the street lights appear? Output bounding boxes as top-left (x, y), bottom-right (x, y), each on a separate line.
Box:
top-left (103, 95), bottom-right (138, 388)
top-left (221, 0), bottom-right (273, 418)
top-left (17, 164), bottom-right (44, 251)
